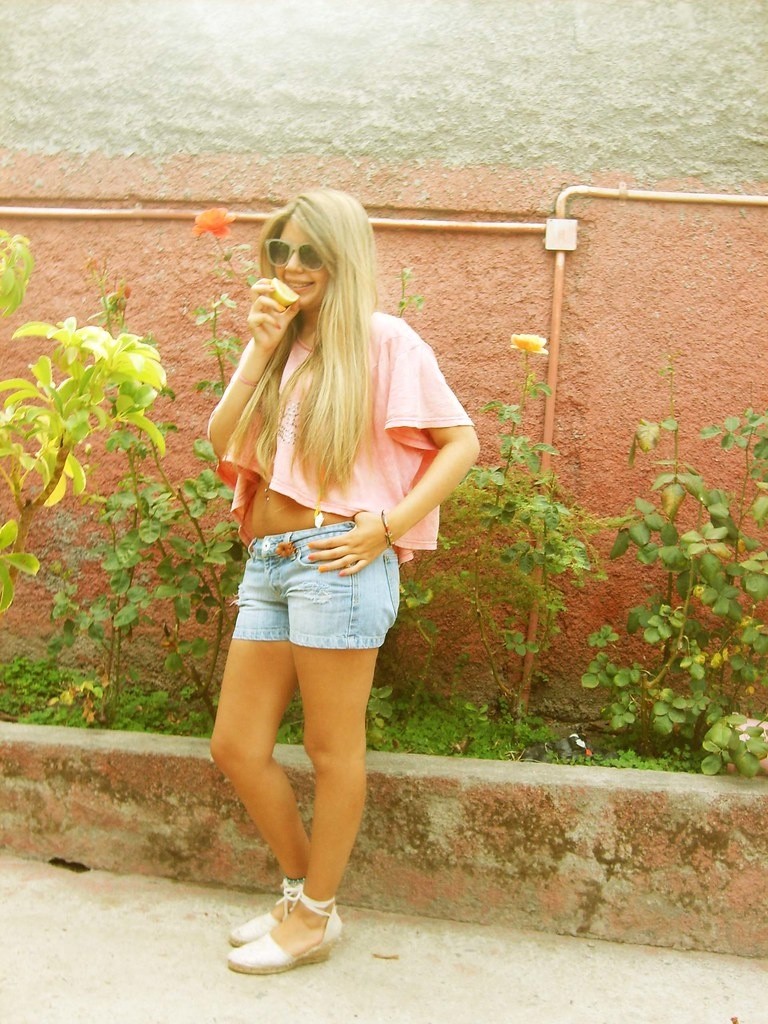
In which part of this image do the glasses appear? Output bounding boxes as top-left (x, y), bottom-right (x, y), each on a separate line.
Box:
top-left (265, 238), bottom-right (325, 271)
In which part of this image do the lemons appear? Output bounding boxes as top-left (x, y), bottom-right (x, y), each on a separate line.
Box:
top-left (270, 277), bottom-right (300, 307)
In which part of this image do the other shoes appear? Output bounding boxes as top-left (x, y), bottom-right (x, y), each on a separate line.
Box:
top-left (229, 878), bottom-right (305, 947)
top-left (228, 891), bottom-right (342, 975)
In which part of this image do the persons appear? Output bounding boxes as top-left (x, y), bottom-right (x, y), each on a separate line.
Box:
top-left (207, 188), bottom-right (480, 974)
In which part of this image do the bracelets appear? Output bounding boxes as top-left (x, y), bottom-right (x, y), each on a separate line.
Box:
top-left (381, 510), bottom-right (393, 547)
top-left (238, 372), bottom-right (257, 386)
